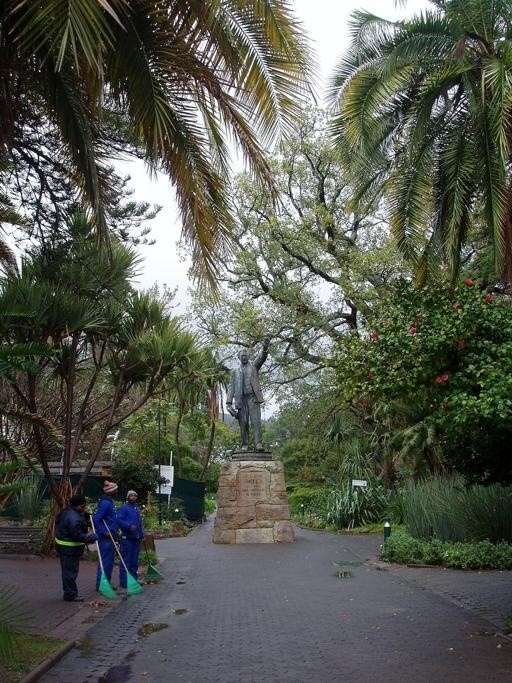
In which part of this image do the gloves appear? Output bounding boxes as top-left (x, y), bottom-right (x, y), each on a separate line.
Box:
top-left (93, 533), bottom-right (100, 543)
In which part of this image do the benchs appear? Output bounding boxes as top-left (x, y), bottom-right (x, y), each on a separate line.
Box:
top-left (0, 526), bottom-right (46, 544)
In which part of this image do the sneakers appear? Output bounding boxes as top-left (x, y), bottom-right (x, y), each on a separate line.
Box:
top-left (120, 582), bottom-right (127, 588)
top-left (111, 583), bottom-right (118, 590)
top-left (71, 596), bottom-right (84, 601)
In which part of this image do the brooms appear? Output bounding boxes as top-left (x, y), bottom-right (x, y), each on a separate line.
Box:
top-left (139, 526), bottom-right (166, 583)
top-left (103, 518), bottom-right (143, 595)
top-left (88, 514), bottom-right (118, 601)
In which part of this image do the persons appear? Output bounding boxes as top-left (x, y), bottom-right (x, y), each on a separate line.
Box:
top-left (54, 494), bottom-right (102, 600)
top-left (225, 336), bottom-right (270, 452)
top-left (117, 490), bottom-right (143, 588)
top-left (92, 480), bottom-right (119, 590)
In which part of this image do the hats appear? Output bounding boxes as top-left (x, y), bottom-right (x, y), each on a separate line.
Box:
top-left (69, 494), bottom-right (86, 506)
top-left (102, 481), bottom-right (118, 493)
top-left (127, 490), bottom-right (137, 499)
